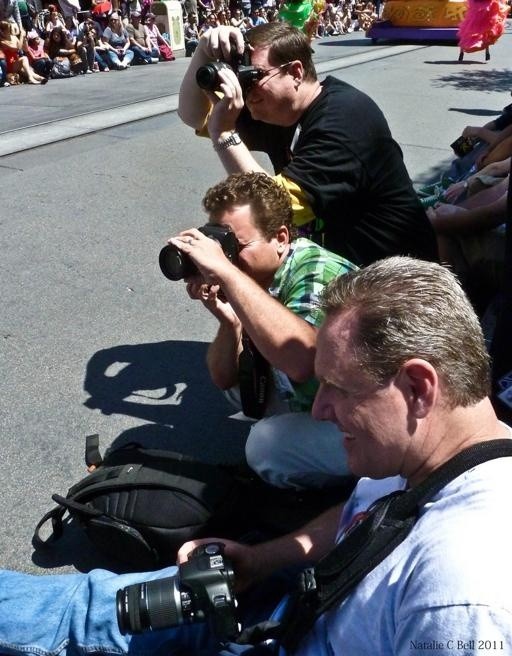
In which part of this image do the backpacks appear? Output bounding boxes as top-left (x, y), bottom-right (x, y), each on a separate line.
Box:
top-left (33, 433), bottom-right (259, 572)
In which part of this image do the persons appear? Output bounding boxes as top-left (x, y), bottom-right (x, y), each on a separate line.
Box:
top-left (0, 26), bottom-right (512, 655)
top-left (1, 0), bottom-right (512, 83)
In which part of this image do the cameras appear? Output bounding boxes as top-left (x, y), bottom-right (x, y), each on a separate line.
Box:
top-left (196, 40), bottom-right (252, 96)
top-left (115, 542), bottom-right (243, 643)
top-left (157, 223), bottom-right (237, 282)
